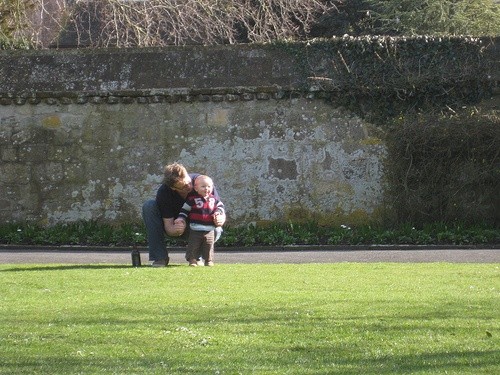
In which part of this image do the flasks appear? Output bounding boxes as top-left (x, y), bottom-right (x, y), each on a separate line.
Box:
top-left (132, 246), bottom-right (141, 266)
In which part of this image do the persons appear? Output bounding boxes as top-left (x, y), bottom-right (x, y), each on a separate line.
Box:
top-left (174, 175), bottom-right (225, 267)
top-left (142, 162), bottom-right (227, 267)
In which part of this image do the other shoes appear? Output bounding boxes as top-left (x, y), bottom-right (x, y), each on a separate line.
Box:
top-left (207, 261), bottom-right (214, 267)
top-left (196, 257), bottom-right (205, 266)
top-left (151, 260), bottom-right (168, 267)
top-left (189, 260), bottom-right (197, 266)
